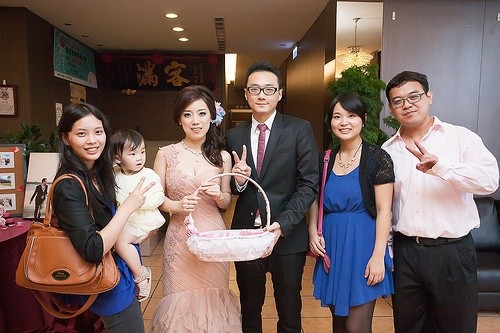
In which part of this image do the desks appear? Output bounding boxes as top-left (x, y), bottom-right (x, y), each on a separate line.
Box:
top-left (0, 218), bottom-right (104, 333)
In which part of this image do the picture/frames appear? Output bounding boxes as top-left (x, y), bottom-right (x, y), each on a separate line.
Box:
top-left (0, 83), bottom-right (19, 118)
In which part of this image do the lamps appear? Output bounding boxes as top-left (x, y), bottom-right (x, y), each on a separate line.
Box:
top-left (338, 18), bottom-right (373, 67)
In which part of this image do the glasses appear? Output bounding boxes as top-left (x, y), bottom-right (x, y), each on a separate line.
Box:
top-left (391, 92), bottom-right (426, 108)
top-left (247, 87), bottom-right (279, 95)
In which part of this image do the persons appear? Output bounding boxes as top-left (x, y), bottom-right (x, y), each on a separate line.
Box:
top-left (51, 102), bottom-right (155, 333)
top-left (225, 63), bottom-right (319, 333)
top-left (111, 129), bottom-right (166, 302)
top-left (380, 71), bottom-right (499, 333)
top-left (29, 177), bottom-right (48, 219)
top-left (153, 85), bottom-right (243, 333)
top-left (308, 93), bottom-right (395, 333)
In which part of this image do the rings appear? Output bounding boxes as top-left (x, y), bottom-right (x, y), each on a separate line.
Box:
top-left (186, 204), bottom-right (189, 209)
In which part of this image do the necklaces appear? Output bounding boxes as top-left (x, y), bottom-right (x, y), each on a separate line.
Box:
top-left (338, 143), bottom-right (362, 169)
top-left (182, 139), bottom-right (203, 162)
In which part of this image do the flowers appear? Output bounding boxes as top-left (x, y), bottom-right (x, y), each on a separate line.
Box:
top-left (211, 101), bottom-right (227, 127)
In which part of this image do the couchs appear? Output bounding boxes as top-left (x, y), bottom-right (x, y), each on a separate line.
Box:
top-left (470, 198), bottom-right (500, 311)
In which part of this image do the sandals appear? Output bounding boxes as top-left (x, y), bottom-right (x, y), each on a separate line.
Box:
top-left (134, 266), bottom-right (151, 302)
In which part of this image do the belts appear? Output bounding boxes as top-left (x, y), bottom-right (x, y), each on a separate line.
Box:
top-left (416, 236), bottom-right (463, 246)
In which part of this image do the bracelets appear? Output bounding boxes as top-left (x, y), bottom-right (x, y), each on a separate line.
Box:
top-left (215, 195), bottom-right (224, 204)
top-left (169, 201), bottom-right (174, 216)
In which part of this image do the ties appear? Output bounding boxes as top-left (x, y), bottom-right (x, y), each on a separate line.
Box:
top-left (255, 123), bottom-right (269, 215)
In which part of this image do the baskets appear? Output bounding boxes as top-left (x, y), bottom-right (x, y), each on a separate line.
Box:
top-left (189, 173), bottom-right (275, 263)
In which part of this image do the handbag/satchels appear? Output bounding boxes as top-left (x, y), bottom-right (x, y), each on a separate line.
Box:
top-left (16, 173), bottom-right (120, 295)
top-left (306, 234), bottom-right (323, 260)
